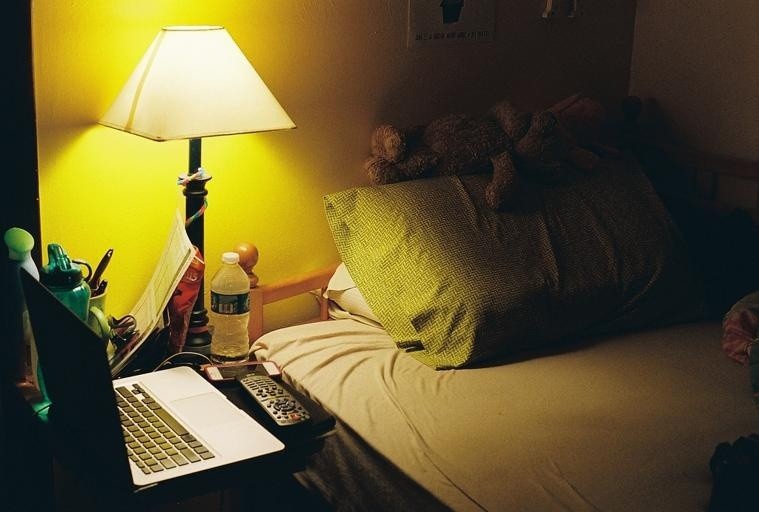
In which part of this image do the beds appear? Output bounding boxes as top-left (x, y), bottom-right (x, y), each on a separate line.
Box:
top-left (249, 278), bottom-right (759, 511)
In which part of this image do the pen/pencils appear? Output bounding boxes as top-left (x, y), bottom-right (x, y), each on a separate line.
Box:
top-left (107, 316), bottom-right (142, 370)
top-left (90, 248), bottom-right (114, 298)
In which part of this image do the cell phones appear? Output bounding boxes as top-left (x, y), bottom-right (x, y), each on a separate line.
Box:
top-left (205, 360), bottom-right (283, 384)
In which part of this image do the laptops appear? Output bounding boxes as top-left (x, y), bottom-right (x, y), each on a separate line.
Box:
top-left (19, 266), bottom-right (286, 492)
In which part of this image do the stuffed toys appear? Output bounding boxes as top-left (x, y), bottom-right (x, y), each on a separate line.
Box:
top-left (362, 101), bottom-right (620, 210)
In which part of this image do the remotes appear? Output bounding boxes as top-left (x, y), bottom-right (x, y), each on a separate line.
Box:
top-left (240, 375), bottom-right (313, 428)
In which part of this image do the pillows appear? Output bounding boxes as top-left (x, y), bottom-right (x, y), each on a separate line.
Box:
top-left (321, 162), bottom-right (707, 368)
top-left (326, 260), bottom-right (379, 325)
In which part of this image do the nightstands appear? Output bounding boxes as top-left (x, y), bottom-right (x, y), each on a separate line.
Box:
top-left (7, 345), bottom-right (338, 504)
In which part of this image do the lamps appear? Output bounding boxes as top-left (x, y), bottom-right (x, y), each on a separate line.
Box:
top-left (96, 26), bottom-right (295, 360)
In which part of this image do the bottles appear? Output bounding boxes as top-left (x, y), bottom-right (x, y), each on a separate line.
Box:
top-left (211, 252), bottom-right (252, 364)
top-left (35, 244), bottom-right (91, 399)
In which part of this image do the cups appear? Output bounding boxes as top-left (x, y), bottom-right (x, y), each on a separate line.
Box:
top-left (86, 291), bottom-right (107, 342)
